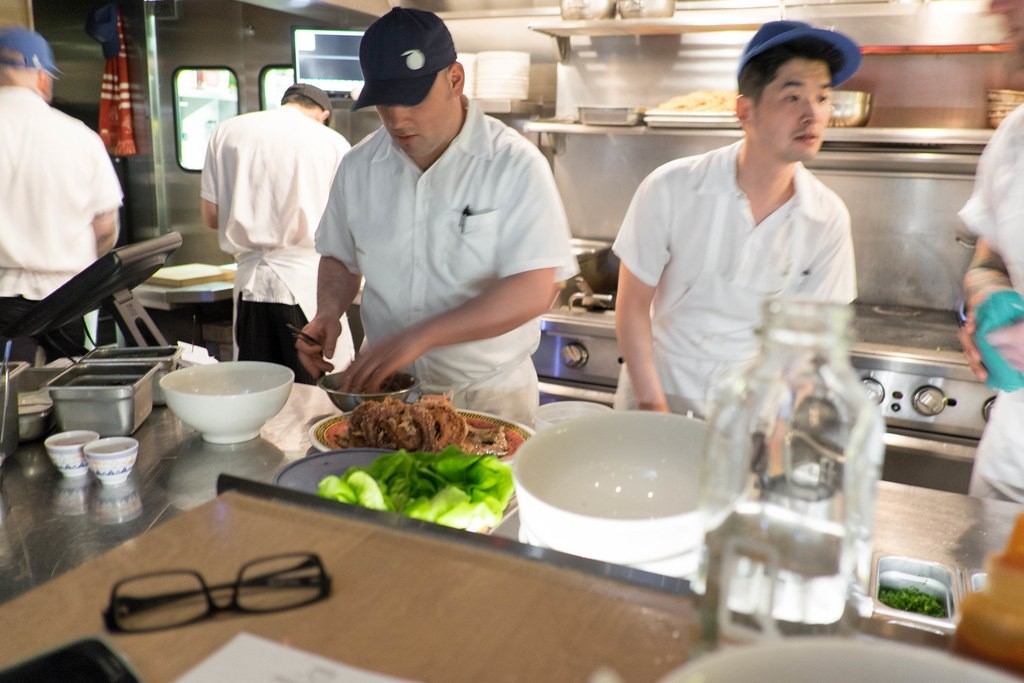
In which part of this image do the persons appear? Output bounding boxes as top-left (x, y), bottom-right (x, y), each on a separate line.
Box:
top-left (954, 101), bottom-right (1024, 508)
top-left (200, 83), bottom-right (355, 385)
top-left (297, 4), bottom-right (580, 432)
top-left (0, 25), bottom-right (123, 368)
top-left (612, 18), bottom-right (864, 439)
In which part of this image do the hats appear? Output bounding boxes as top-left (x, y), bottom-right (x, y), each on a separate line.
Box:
top-left (0, 27), bottom-right (64, 80)
top-left (281, 83), bottom-right (332, 128)
top-left (351, 6), bottom-right (458, 113)
top-left (85, 1), bottom-right (120, 59)
top-left (736, 20), bottom-right (864, 88)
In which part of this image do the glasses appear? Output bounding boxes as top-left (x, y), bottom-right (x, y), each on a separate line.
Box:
top-left (102, 551), bottom-right (330, 635)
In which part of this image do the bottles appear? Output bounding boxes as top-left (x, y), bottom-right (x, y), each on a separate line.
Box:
top-left (947, 513), bottom-right (1024, 680)
top-left (688, 295), bottom-right (889, 658)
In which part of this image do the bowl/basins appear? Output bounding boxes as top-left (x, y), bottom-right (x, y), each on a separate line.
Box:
top-left (82, 436), bottom-right (139, 485)
top-left (158, 360), bottom-right (295, 443)
top-left (510, 407), bottom-right (749, 562)
top-left (318, 369), bottom-right (419, 412)
top-left (49, 478), bottom-right (97, 516)
top-left (985, 88), bottom-right (1024, 129)
top-left (559, 1), bottom-right (674, 19)
top-left (89, 485), bottom-right (144, 524)
top-left (45, 428), bottom-right (101, 477)
top-left (654, 635), bottom-right (1024, 683)
top-left (826, 90), bottom-right (873, 127)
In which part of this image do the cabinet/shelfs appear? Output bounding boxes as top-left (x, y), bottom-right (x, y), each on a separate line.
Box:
top-left (514, 14), bottom-right (1024, 144)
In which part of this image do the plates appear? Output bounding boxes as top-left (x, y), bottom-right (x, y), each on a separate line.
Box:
top-left (310, 408), bottom-right (536, 465)
top-left (274, 447), bottom-right (399, 495)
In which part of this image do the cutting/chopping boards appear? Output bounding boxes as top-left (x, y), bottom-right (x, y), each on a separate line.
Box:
top-left (144, 262), bottom-right (236, 287)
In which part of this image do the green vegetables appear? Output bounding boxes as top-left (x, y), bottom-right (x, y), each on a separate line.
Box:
top-left (878, 585), bottom-right (947, 619)
top-left (317, 446), bottom-right (514, 535)
